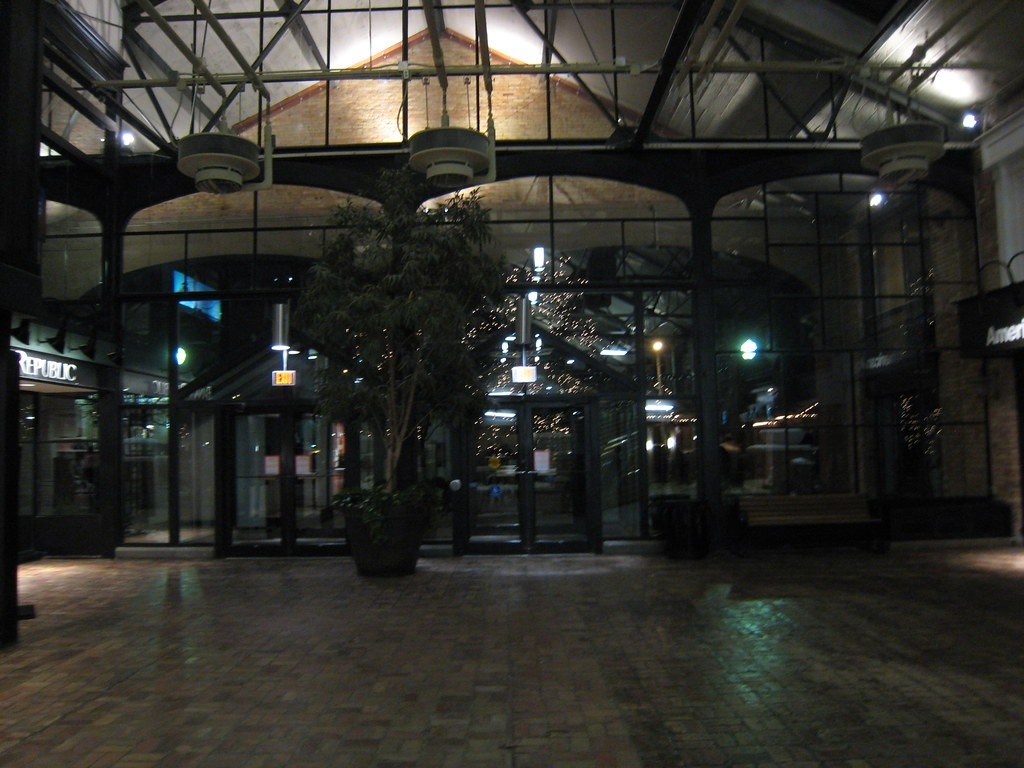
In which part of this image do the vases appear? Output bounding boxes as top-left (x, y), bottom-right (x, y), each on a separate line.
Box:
top-left (654, 499), bottom-right (710, 560)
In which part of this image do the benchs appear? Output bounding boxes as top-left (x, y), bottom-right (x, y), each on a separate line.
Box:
top-left (732, 488), bottom-right (895, 556)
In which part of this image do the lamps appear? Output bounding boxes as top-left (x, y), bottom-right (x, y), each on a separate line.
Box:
top-left (516, 297), bottom-right (531, 349)
top-left (270, 302), bottom-right (291, 352)
top-left (10, 318), bottom-right (126, 366)
top-left (1007, 248), bottom-right (1024, 307)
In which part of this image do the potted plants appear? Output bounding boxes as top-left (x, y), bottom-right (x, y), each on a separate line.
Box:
top-left (309, 168), bottom-right (530, 577)
